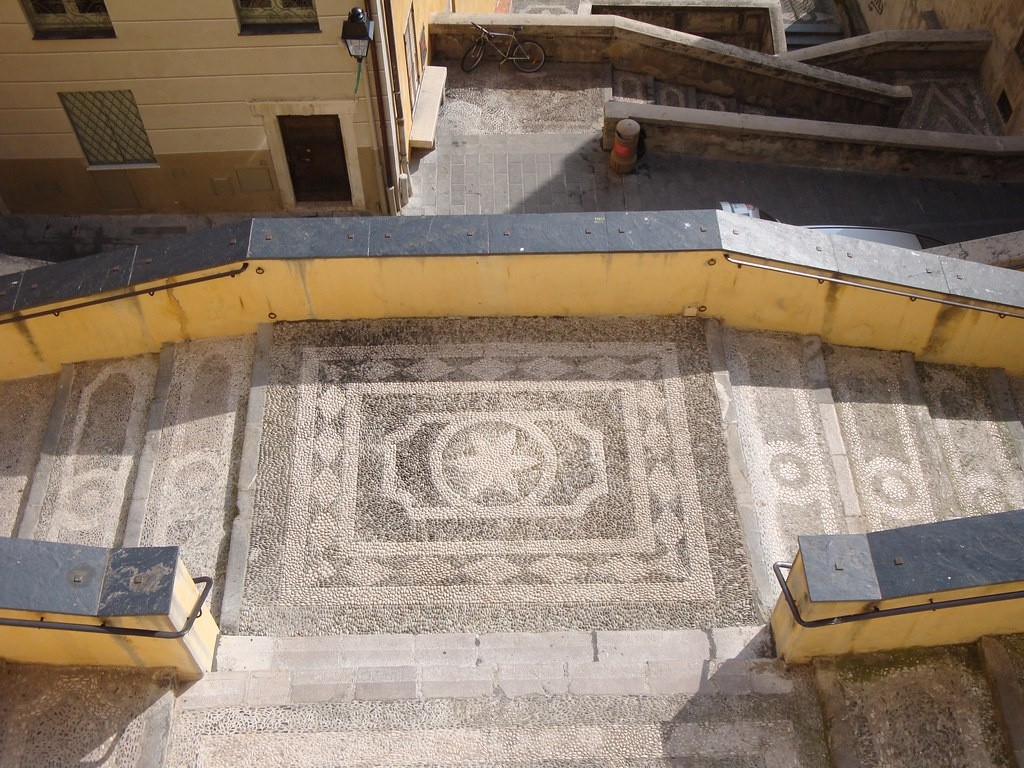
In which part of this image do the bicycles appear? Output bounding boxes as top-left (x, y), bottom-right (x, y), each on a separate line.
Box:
top-left (461, 21), bottom-right (546, 74)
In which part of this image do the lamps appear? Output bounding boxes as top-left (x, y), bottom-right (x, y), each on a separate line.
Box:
top-left (341, 7), bottom-right (375, 63)
